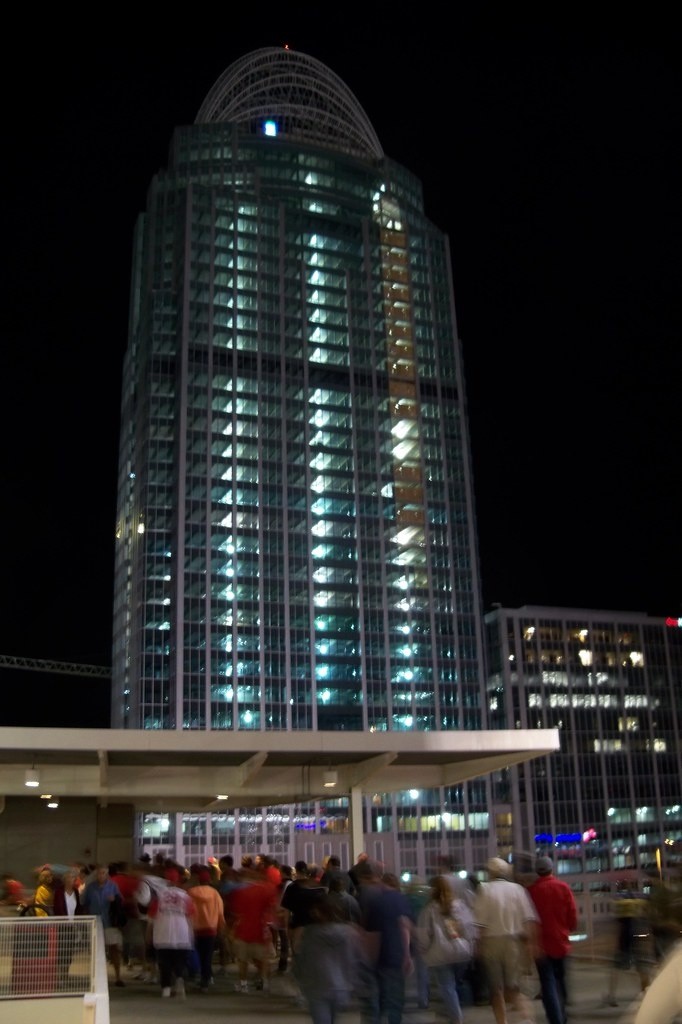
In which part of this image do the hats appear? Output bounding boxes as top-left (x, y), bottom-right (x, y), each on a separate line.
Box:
top-left (487, 857), bottom-right (508, 878)
top-left (535, 856), bottom-right (554, 874)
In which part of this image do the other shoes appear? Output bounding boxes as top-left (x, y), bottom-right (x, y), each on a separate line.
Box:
top-left (202, 977), bottom-right (213, 992)
top-left (255, 979), bottom-right (263, 991)
top-left (162, 987), bottom-right (171, 998)
top-left (602, 994), bottom-right (618, 1007)
top-left (176, 977), bottom-right (184, 995)
top-left (132, 972), bottom-right (150, 980)
top-left (143, 976), bottom-right (157, 984)
top-left (115, 980), bottom-right (124, 987)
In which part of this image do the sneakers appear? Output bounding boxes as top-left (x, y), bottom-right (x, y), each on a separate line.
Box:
top-left (233, 984), bottom-right (248, 993)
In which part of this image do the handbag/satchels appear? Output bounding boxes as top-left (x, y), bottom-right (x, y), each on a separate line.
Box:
top-left (137, 880), bottom-right (156, 915)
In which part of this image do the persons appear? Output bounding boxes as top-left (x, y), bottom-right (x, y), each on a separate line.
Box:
top-left (632, 945), bottom-right (682, 1024)
top-left (0, 837), bottom-right (579, 1024)
